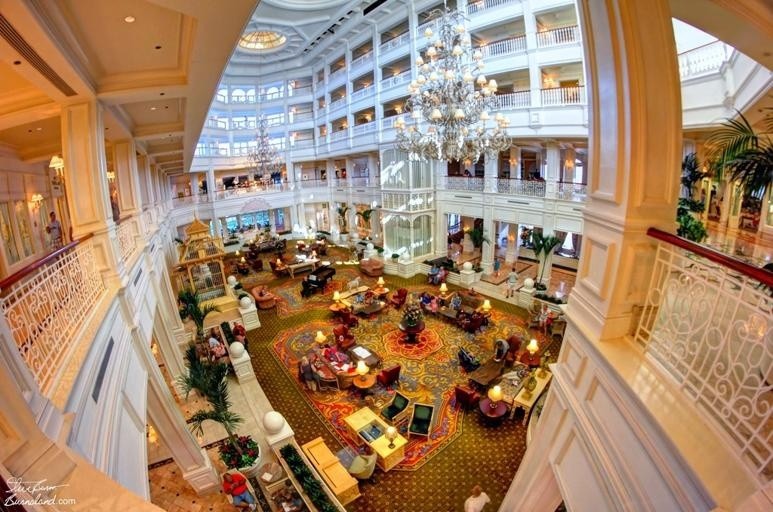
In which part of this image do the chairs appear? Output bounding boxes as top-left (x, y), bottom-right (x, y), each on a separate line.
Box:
top-left (552, 310), bottom-right (567, 338)
top-left (349, 452), bottom-right (377, 480)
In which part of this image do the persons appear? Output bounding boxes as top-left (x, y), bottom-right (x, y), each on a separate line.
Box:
top-left (535, 304), bottom-right (550, 336)
top-left (463, 170), bottom-right (472, 177)
top-left (322, 344), bottom-right (350, 362)
top-left (423, 292), bottom-right (462, 313)
top-left (300, 356), bottom-right (317, 392)
top-left (428, 263), bottom-right (445, 285)
top-left (45, 211), bottom-right (63, 253)
top-left (222, 473), bottom-right (257, 512)
top-left (527, 173), bottom-right (544, 182)
top-left (110, 196), bottom-right (120, 224)
top-left (464, 485), bottom-right (490, 512)
top-left (356, 288), bottom-right (375, 305)
top-left (205, 322), bottom-right (248, 360)
top-left (494, 339), bottom-right (509, 359)
top-left (505, 268), bottom-right (518, 298)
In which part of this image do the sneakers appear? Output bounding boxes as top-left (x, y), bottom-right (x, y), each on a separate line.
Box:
top-left (249, 504), bottom-right (256, 510)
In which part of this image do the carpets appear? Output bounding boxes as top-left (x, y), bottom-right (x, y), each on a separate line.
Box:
top-left (224, 241), bottom-right (555, 472)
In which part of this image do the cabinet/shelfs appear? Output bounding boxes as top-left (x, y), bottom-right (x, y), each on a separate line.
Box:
top-left (301, 437), bottom-right (361, 508)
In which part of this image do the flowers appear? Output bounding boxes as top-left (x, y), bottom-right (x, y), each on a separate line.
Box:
top-left (217, 434), bottom-right (259, 472)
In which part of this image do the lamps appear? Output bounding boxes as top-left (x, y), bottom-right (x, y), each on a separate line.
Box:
top-left (32, 193), bottom-right (44, 210)
top-left (244, 40), bottom-right (286, 181)
top-left (49, 154), bottom-right (65, 188)
top-left (393, 0), bottom-right (513, 166)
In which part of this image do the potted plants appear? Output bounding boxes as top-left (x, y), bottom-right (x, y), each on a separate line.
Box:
top-left (464, 229), bottom-right (492, 281)
top-left (175, 341), bottom-right (262, 480)
top-left (177, 289), bottom-right (222, 341)
top-left (338, 204), bottom-right (377, 244)
top-left (678, 152), bottom-right (705, 222)
top-left (525, 229), bottom-right (563, 296)
